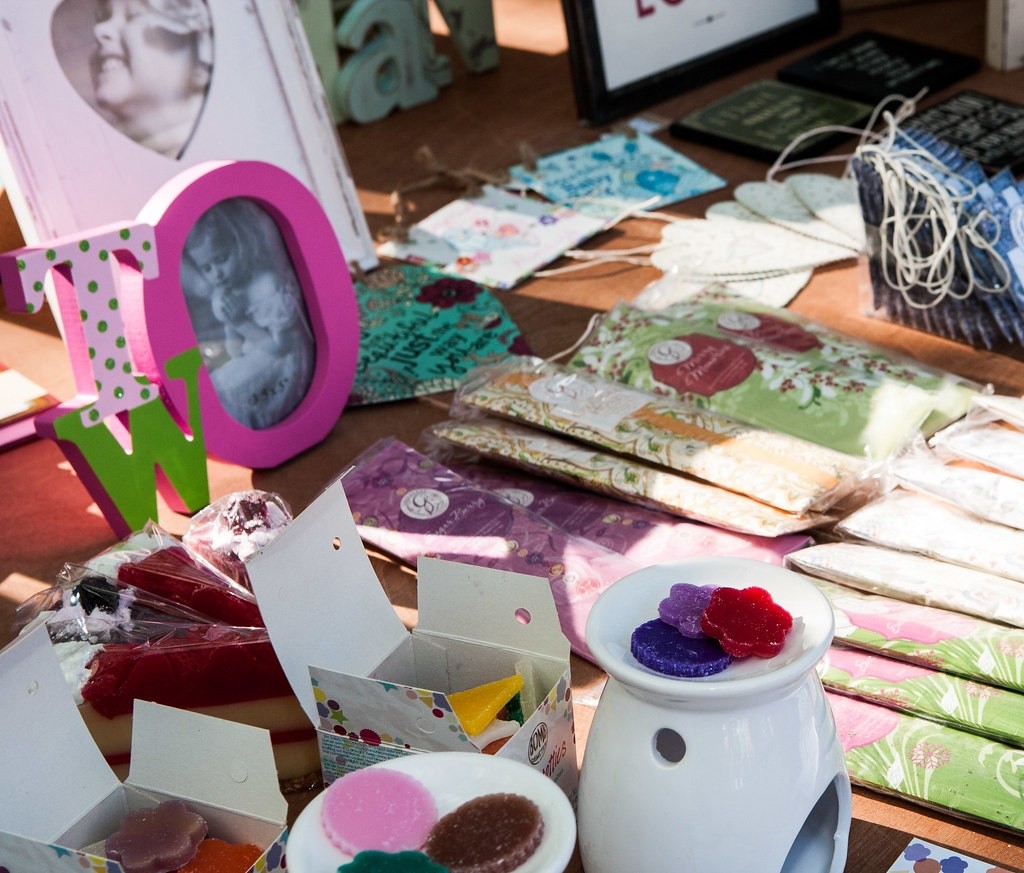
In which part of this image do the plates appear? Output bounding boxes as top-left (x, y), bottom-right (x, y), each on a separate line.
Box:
top-left (287, 752), bottom-right (576, 873)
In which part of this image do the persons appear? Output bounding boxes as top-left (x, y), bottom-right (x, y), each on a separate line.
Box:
top-left (92, 0), bottom-right (212, 157)
top-left (183, 200), bottom-right (315, 428)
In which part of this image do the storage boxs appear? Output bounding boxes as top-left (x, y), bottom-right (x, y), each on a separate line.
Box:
top-left (0, 611), bottom-right (289, 873)
top-left (244, 466), bottom-right (579, 816)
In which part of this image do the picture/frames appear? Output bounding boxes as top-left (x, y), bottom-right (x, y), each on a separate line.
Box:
top-left (560, 0), bottom-right (842, 128)
top-left (122, 158), bottom-right (360, 469)
top-left (0, 0), bottom-right (380, 278)
